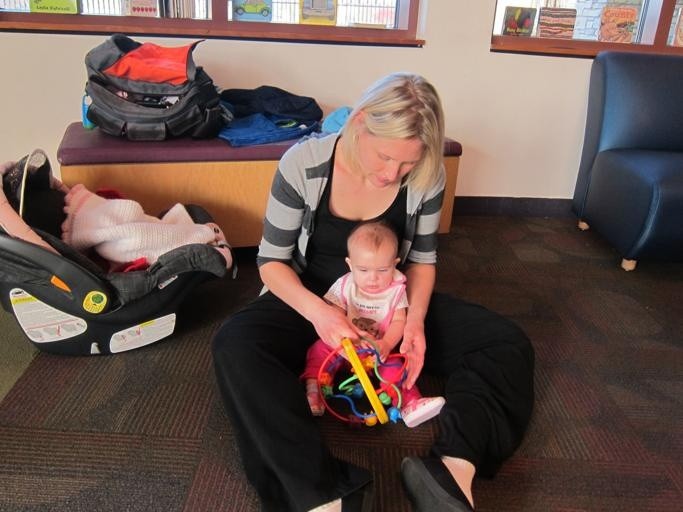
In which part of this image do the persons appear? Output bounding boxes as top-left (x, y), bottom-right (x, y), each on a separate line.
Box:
top-left (209, 70), bottom-right (534, 512)
top-left (303, 222), bottom-right (447, 431)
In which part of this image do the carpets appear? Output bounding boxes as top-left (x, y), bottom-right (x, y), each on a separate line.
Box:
top-left (0, 217), bottom-right (682, 511)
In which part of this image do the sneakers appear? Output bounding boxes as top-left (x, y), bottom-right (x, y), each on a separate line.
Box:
top-left (399, 454), bottom-right (476, 512)
top-left (305, 390), bottom-right (327, 416)
top-left (400, 396), bottom-right (445, 428)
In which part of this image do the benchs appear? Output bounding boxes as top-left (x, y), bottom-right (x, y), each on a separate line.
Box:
top-left (56, 116), bottom-right (461, 247)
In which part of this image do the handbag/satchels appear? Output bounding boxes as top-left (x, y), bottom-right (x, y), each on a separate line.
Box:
top-left (83, 34), bottom-right (233, 143)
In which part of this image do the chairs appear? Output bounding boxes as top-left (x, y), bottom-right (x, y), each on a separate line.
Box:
top-left (0, 160), bottom-right (240, 357)
top-left (572, 51), bottom-right (683, 271)
